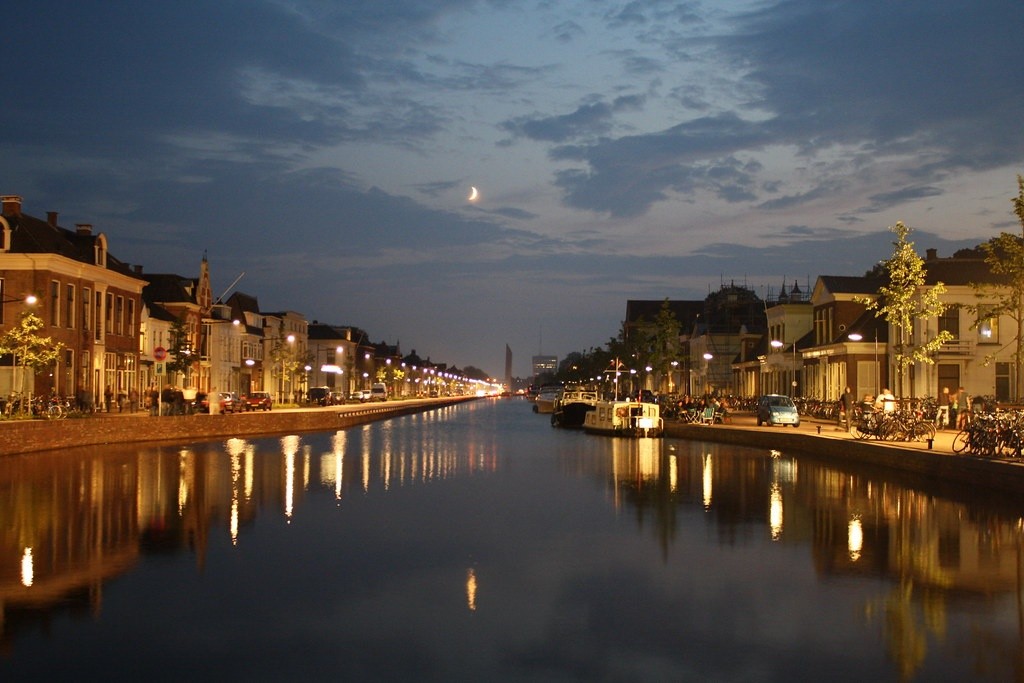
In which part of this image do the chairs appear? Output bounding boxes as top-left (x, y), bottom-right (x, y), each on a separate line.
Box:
top-left (677, 407), bottom-right (727, 426)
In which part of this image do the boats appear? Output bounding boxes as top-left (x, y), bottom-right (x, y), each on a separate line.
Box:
top-left (549, 389), bottom-right (600, 429)
top-left (533, 391), bottom-right (562, 415)
top-left (583, 356), bottom-right (665, 438)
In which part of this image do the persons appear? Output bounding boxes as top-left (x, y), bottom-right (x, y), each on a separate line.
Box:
top-left (839, 386), bottom-right (854, 433)
top-left (195, 386), bottom-right (223, 415)
top-left (676, 395), bottom-right (732, 425)
top-left (161, 385), bottom-right (184, 416)
top-left (859, 387), bottom-right (897, 428)
top-left (955, 387), bottom-right (971, 431)
top-left (76, 384), bottom-right (93, 415)
top-left (934, 385), bottom-right (954, 431)
top-left (104, 385), bottom-right (114, 413)
top-left (130, 388), bottom-right (139, 414)
top-left (149, 386), bottom-right (159, 417)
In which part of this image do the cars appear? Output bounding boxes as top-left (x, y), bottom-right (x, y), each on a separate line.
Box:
top-left (349, 389), bottom-right (373, 403)
top-left (328, 392), bottom-right (346, 407)
top-left (755, 394), bottom-right (801, 428)
top-left (244, 391), bottom-right (272, 411)
top-left (197, 391), bottom-right (244, 414)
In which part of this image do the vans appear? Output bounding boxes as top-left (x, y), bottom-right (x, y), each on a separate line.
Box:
top-left (372, 383), bottom-right (388, 401)
top-left (306, 387), bottom-right (331, 406)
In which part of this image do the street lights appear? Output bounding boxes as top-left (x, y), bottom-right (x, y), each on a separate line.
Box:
top-left (0, 295), bottom-right (38, 305)
top-left (703, 350), bottom-right (728, 399)
top-left (197, 318), bottom-right (240, 396)
top-left (258, 333), bottom-right (370, 398)
top-left (770, 337), bottom-right (797, 396)
top-left (645, 360), bottom-right (680, 394)
top-left (849, 326), bottom-right (881, 401)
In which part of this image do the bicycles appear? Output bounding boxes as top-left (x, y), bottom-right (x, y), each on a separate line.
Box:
top-left (12, 393), bottom-right (68, 420)
top-left (953, 410), bottom-right (1024, 455)
top-left (724, 394), bottom-right (936, 441)
